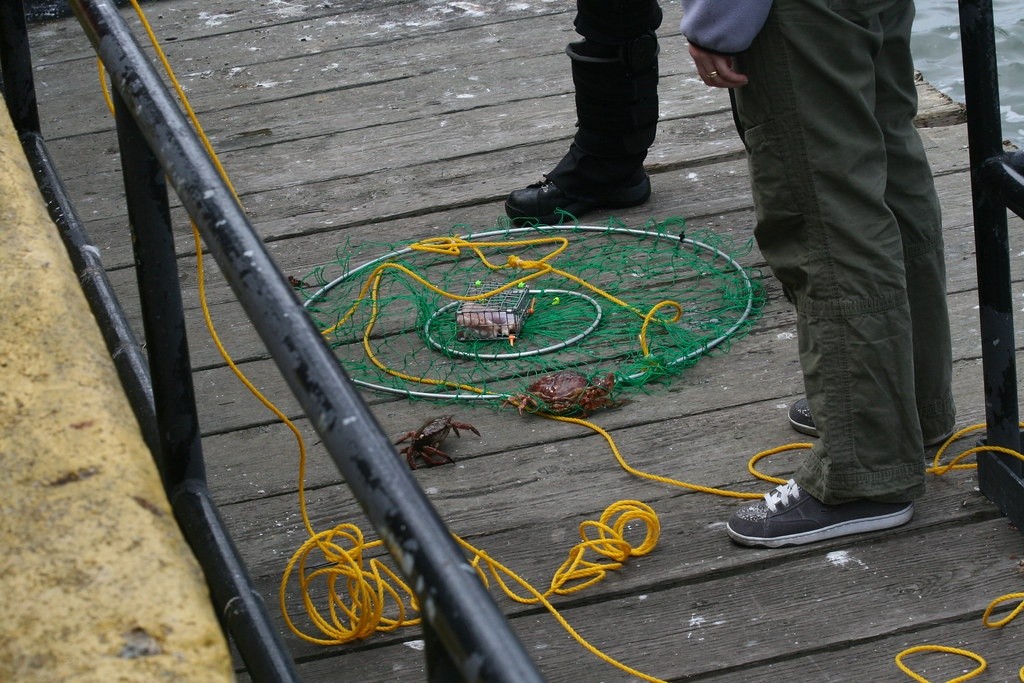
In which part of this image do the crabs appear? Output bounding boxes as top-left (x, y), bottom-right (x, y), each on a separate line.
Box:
top-left (393, 413), bottom-right (482, 470)
top-left (499, 369), bottom-right (624, 419)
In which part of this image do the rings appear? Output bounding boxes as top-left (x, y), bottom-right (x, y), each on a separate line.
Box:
top-left (706, 71), bottom-right (718, 79)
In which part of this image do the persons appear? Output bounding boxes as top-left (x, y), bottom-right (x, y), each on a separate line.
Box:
top-left (680, 0), bottom-right (955, 549)
top-left (505, 0), bottom-right (744, 228)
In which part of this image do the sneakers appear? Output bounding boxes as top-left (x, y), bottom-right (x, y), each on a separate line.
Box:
top-left (504, 171), bottom-right (652, 227)
top-left (788, 396), bottom-right (953, 447)
top-left (724, 476), bottom-right (915, 549)
